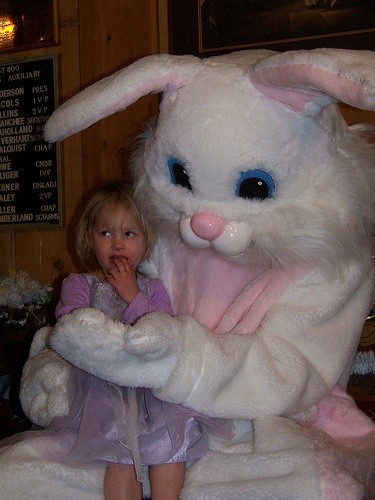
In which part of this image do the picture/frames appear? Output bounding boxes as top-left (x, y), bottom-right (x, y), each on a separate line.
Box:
top-left (197, 0), bottom-right (375, 52)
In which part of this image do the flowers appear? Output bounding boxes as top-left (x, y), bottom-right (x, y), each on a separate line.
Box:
top-left (0, 269), bottom-right (54, 326)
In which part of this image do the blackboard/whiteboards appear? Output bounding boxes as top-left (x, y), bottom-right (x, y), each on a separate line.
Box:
top-left (0, 51), bottom-right (66, 232)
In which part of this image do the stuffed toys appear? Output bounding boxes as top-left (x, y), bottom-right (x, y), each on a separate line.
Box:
top-left (0, 47), bottom-right (374, 500)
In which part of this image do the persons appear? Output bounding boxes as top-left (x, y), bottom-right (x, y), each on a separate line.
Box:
top-left (55, 183), bottom-right (236, 500)
